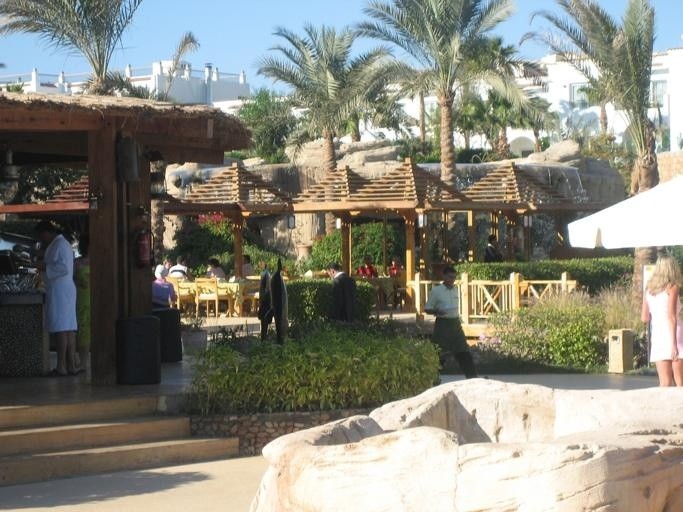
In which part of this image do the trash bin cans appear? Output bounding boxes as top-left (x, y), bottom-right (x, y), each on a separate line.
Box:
top-left (608, 328), bottom-right (634, 374)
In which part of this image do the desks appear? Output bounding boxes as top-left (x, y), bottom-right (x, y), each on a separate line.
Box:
top-left (246, 275), bottom-right (289, 281)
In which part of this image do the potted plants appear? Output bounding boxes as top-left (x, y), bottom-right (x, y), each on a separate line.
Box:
top-left (182, 302), bottom-right (207, 355)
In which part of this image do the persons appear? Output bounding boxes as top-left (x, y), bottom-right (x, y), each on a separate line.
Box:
top-left (326, 263), bottom-right (359, 325)
top-left (167, 255), bottom-right (195, 318)
top-left (356, 254), bottom-right (377, 276)
top-left (639, 252), bottom-right (682, 388)
top-left (387, 256), bottom-right (404, 278)
top-left (206, 257), bottom-right (226, 278)
top-left (10, 220), bottom-right (77, 374)
top-left (151, 264), bottom-right (177, 309)
top-left (484, 233), bottom-right (502, 262)
top-left (163, 257), bottom-right (171, 274)
top-left (72, 231), bottom-right (90, 374)
top-left (423, 265), bottom-right (476, 379)
top-left (241, 255), bottom-right (251, 274)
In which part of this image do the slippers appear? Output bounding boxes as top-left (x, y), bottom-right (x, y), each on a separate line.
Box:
top-left (45, 369), bottom-right (67, 376)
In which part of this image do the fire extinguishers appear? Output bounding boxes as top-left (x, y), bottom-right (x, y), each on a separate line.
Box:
top-left (135, 220), bottom-right (150, 269)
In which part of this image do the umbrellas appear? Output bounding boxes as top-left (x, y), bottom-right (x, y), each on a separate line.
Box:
top-left (566, 171), bottom-right (683, 250)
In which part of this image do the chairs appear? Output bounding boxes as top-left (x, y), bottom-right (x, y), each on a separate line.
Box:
top-left (164, 276), bottom-right (260, 320)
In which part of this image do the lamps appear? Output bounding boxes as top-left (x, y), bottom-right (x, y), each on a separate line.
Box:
top-left (522, 212), bottom-right (534, 229)
top-left (88, 192), bottom-right (104, 212)
top-left (336, 218), bottom-right (341, 230)
top-left (150, 172), bottom-right (165, 199)
top-left (418, 214), bottom-right (428, 230)
